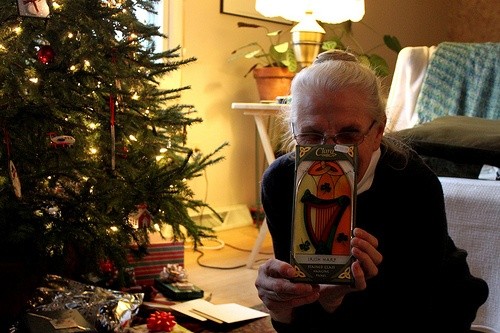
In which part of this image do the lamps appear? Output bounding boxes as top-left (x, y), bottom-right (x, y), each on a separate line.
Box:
top-left (255, 0), bottom-right (366, 70)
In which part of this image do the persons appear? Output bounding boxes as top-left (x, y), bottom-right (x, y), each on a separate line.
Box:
top-left (255, 50), bottom-right (489, 333)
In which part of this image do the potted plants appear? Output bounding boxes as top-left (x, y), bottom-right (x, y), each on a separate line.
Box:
top-left (232, 18), bottom-right (402, 104)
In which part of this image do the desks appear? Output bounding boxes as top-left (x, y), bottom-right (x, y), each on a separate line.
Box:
top-left (231, 103), bottom-right (296, 269)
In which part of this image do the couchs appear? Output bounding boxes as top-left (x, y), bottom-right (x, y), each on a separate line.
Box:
top-left (384, 41), bottom-right (500, 333)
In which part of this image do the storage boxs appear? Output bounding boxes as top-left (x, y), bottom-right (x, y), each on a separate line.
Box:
top-left (153, 276), bottom-right (203, 302)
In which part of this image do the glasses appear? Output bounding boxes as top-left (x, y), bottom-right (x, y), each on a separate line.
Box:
top-left (291, 119), bottom-right (377, 147)
